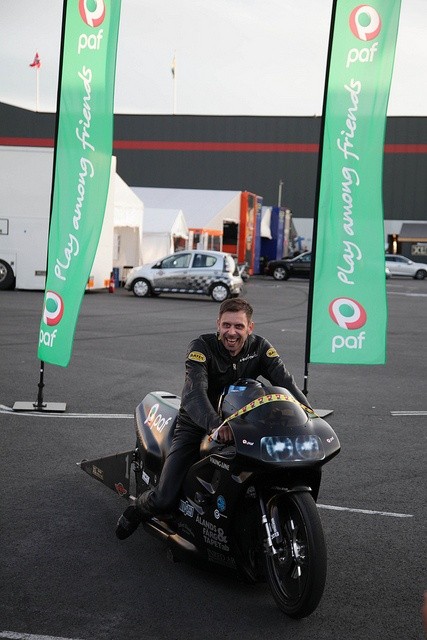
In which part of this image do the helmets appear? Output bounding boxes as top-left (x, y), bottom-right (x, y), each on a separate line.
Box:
top-left (222, 378), bottom-right (272, 421)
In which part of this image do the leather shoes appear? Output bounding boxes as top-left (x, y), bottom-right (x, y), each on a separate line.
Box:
top-left (116, 493), bottom-right (144, 540)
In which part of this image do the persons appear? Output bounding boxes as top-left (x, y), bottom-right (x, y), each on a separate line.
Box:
top-left (112, 297), bottom-right (314, 542)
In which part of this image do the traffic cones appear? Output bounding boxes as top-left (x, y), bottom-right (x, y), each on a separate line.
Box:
top-left (108, 272), bottom-right (115, 293)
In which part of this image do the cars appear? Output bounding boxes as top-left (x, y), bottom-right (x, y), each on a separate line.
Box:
top-left (124, 248), bottom-right (243, 303)
top-left (385, 266), bottom-right (392, 279)
top-left (385, 254), bottom-right (427, 279)
top-left (265, 251), bottom-right (313, 280)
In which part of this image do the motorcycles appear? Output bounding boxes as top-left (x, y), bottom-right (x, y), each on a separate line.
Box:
top-left (74, 374), bottom-right (343, 619)
